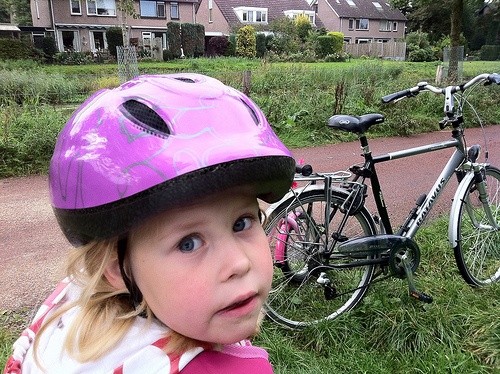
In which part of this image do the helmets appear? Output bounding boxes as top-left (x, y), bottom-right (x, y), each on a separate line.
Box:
top-left (48, 72), bottom-right (295, 247)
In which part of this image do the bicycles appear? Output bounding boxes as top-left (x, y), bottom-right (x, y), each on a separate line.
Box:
top-left (256, 71), bottom-right (500, 332)
top-left (233, 164), bottom-right (320, 282)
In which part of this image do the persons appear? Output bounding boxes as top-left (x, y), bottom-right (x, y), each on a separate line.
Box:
top-left (3, 73), bottom-right (296, 374)
